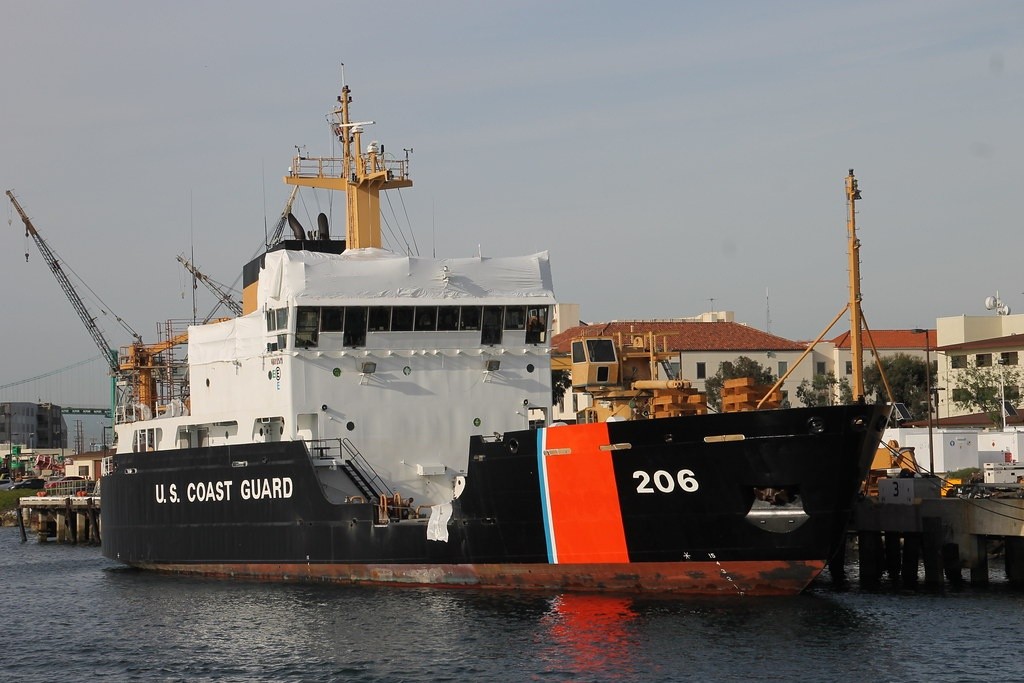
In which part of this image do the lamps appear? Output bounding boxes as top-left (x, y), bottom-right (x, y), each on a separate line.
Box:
top-left (360, 362), bottom-right (376, 385)
top-left (483, 360), bottom-right (500, 382)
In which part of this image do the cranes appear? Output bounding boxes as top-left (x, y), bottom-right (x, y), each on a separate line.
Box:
top-left (6, 187), bottom-right (242, 444)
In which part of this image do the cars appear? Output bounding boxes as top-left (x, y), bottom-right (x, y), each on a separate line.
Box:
top-left (0, 475), bottom-right (96, 494)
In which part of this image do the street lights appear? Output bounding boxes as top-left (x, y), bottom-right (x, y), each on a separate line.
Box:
top-left (104, 426), bottom-right (112, 458)
top-left (9, 433), bottom-right (19, 481)
top-left (911, 327), bottom-right (935, 477)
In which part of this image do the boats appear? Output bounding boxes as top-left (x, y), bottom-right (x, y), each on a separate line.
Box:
top-left (98, 62), bottom-right (896, 601)
